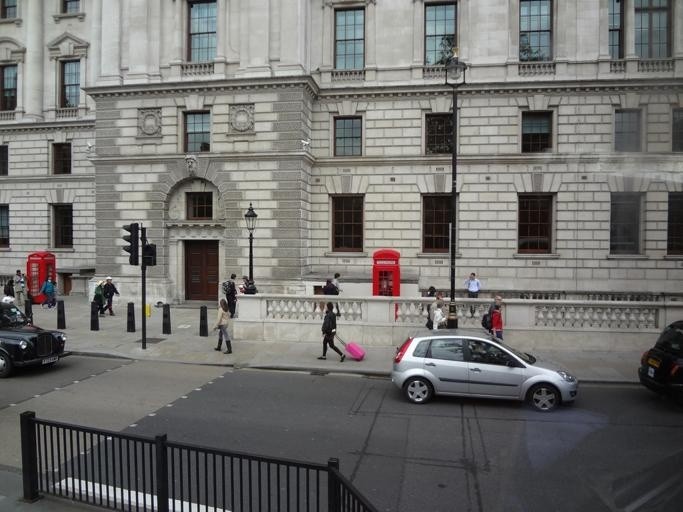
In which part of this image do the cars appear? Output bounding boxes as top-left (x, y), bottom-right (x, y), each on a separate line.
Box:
top-left (391, 328), bottom-right (580, 412)
top-left (638, 320), bottom-right (683, 398)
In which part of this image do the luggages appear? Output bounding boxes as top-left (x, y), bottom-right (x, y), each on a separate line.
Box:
top-left (335, 335), bottom-right (365, 361)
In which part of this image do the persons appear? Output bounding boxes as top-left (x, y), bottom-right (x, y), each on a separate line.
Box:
top-left (464, 272), bottom-right (481, 317)
top-left (473, 295), bottom-right (504, 364)
top-left (223, 274), bottom-right (257, 318)
top-left (4, 270), bottom-right (59, 311)
top-left (324, 279), bottom-right (339, 295)
top-left (426, 287), bottom-right (448, 329)
top-left (93, 280), bottom-right (106, 317)
top-left (103, 276), bottom-right (119, 316)
top-left (317, 302), bottom-right (345, 362)
top-left (332, 273), bottom-right (343, 295)
top-left (213, 299), bottom-right (232, 354)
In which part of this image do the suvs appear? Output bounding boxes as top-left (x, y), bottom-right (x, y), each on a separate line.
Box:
top-left (0, 300), bottom-right (73, 378)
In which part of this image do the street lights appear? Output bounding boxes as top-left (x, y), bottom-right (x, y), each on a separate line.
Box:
top-left (443, 57), bottom-right (468, 328)
top-left (244, 201), bottom-right (258, 294)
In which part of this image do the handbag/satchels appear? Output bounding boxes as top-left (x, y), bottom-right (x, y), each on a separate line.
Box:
top-left (426, 317), bottom-right (433, 329)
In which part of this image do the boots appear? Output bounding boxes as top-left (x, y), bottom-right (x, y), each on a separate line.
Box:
top-left (215, 339), bottom-right (222, 350)
top-left (224, 340), bottom-right (231, 354)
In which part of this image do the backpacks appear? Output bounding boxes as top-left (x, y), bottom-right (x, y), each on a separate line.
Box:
top-left (223, 281), bottom-right (230, 294)
top-left (482, 314), bottom-right (492, 333)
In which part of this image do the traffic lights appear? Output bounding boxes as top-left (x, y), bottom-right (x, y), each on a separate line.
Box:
top-left (122, 223), bottom-right (138, 265)
top-left (142, 244), bottom-right (155, 265)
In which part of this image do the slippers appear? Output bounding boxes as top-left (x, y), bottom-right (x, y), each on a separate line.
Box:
top-left (340, 355), bottom-right (345, 361)
top-left (318, 357), bottom-right (326, 359)
